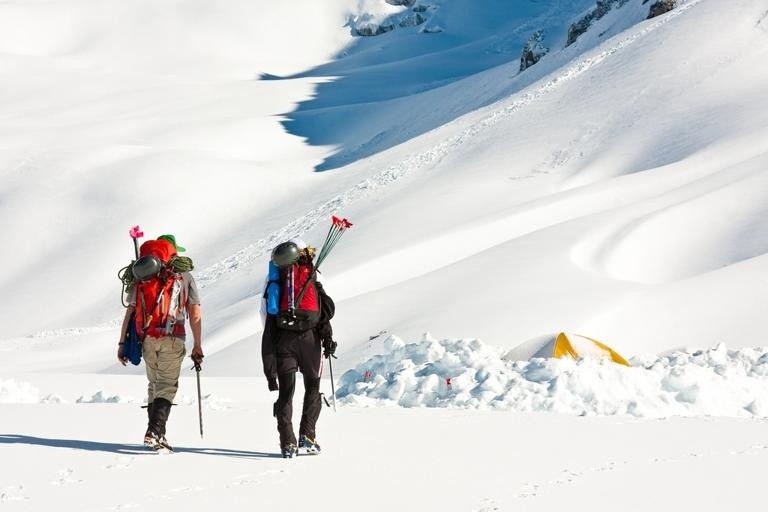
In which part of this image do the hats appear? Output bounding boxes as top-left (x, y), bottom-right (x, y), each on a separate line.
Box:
top-left (157, 235), bottom-right (186, 253)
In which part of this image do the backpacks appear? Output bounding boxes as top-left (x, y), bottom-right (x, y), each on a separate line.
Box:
top-left (136, 275), bottom-right (182, 335)
top-left (274, 260), bottom-right (322, 331)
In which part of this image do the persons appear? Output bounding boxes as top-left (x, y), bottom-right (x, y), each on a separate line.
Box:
top-left (116, 232), bottom-right (205, 453)
top-left (260, 238), bottom-right (337, 459)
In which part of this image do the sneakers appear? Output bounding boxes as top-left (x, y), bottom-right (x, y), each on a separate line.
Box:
top-left (281, 437), bottom-right (298, 459)
top-left (299, 434), bottom-right (321, 456)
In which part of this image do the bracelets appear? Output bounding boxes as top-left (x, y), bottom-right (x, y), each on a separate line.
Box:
top-left (118, 341), bottom-right (126, 347)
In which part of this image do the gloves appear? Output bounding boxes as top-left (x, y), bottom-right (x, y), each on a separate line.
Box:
top-left (324, 338), bottom-right (337, 359)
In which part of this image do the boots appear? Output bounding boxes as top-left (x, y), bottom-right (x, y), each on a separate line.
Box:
top-left (142, 398), bottom-right (174, 454)
